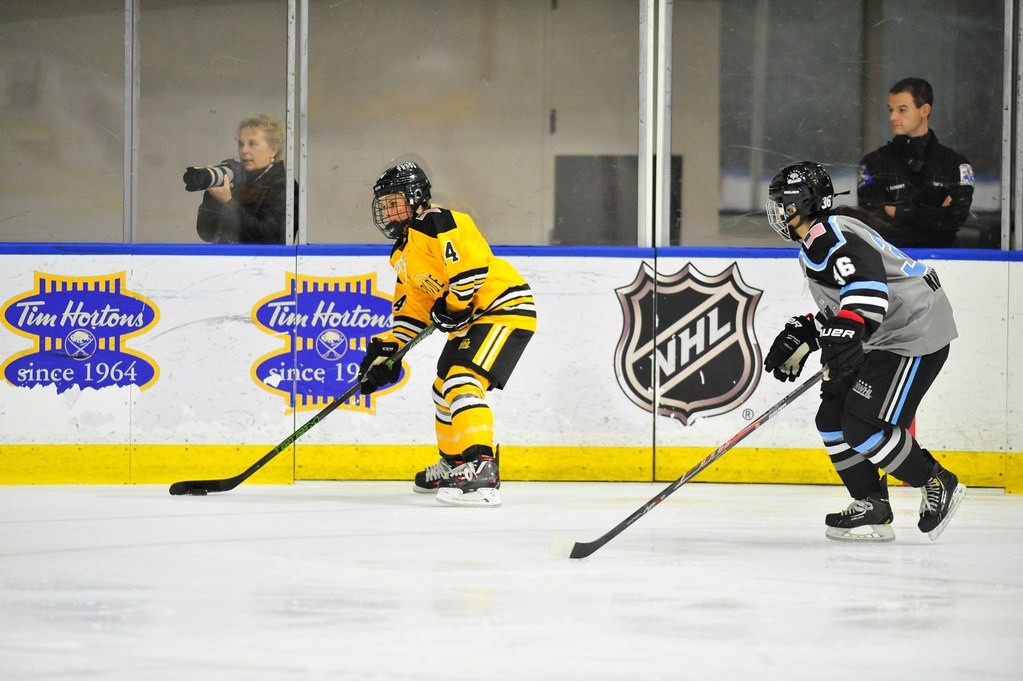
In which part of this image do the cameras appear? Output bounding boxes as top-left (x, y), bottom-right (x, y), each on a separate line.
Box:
top-left (184, 158), bottom-right (243, 191)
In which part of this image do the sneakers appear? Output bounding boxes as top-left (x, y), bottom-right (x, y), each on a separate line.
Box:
top-left (825, 472), bottom-right (896, 542)
top-left (413, 458), bottom-right (463, 493)
top-left (918, 449), bottom-right (968, 541)
top-left (436, 444), bottom-right (502, 506)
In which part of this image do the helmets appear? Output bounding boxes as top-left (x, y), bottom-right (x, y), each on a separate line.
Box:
top-left (766, 161), bottom-right (834, 242)
top-left (371, 161), bottom-right (431, 240)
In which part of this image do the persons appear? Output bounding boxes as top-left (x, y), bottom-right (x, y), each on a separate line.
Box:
top-left (766, 160), bottom-right (967, 543)
top-left (856, 78), bottom-right (975, 248)
top-left (357, 162), bottom-right (536, 507)
top-left (197, 115), bottom-right (300, 243)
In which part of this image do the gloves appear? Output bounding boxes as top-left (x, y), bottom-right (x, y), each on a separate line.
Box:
top-left (358, 338), bottom-right (402, 394)
top-left (764, 315), bottom-right (819, 382)
top-left (430, 291), bottom-right (474, 333)
top-left (818, 309), bottom-right (866, 373)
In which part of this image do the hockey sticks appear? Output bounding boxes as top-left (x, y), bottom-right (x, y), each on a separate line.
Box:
top-left (546, 365), bottom-right (824, 561)
top-left (171, 323), bottom-right (442, 497)
